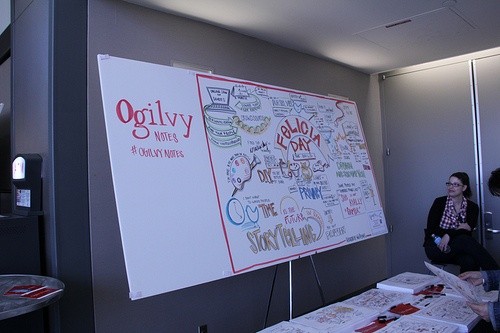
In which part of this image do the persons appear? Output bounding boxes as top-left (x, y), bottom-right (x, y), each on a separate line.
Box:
top-left (459, 169), bottom-right (499, 333)
top-left (422, 172), bottom-right (481, 274)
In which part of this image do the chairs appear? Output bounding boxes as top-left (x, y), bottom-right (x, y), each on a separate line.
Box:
top-left (423, 229), bottom-right (448, 275)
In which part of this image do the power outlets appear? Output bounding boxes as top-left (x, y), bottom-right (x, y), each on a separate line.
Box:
top-left (198, 324), bottom-right (208, 333)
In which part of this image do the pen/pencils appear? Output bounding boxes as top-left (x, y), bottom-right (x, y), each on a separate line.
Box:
top-left (377, 315), bottom-right (398, 323)
top-left (424, 292), bottom-right (446, 299)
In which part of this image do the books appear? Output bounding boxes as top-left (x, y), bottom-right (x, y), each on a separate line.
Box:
top-left (256, 271), bottom-right (499, 333)
top-left (376, 273), bottom-right (441, 296)
top-left (2, 285), bottom-right (62, 300)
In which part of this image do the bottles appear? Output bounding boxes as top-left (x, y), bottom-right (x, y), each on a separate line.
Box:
top-left (431, 234), bottom-right (451, 253)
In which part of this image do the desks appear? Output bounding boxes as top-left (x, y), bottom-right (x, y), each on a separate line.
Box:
top-left (0, 274), bottom-right (65, 318)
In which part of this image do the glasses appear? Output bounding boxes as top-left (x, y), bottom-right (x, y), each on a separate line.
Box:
top-left (446, 182), bottom-right (464, 187)
